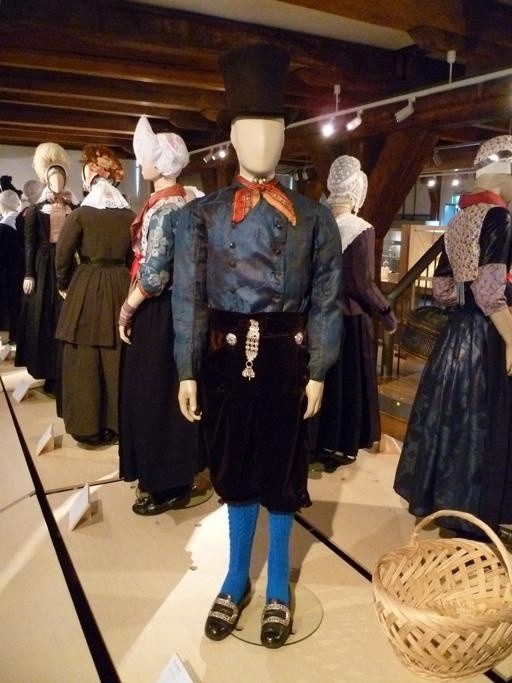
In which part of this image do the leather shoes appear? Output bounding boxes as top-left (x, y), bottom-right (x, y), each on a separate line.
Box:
top-left (206, 574), bottom-right (252, 642)
top-left (131, 493), bottom-right (191, 514)
top-left (72, 429), bottom-right (114, 445)
top-left (259, 599), bottom-right (292, 648)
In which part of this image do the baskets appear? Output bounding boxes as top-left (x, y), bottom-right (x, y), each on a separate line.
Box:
top-left (371, 509), bottom-right (511, 678)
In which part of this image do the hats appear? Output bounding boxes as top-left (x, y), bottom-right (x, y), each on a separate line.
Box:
top-left (34, 143), bottom-right (68, 185)
top-left (324, 155), bottom-right (369, 211)
top-left (81, 145), bottom-right (123, 193)
top-left (474, 134), bottom-right (512, 175)
top-left (133, 115), bottom-right (190, 178)
top-left (216, 41), bottom-right (299, 134)
top-left (0, 190), bottom-right (21, 212)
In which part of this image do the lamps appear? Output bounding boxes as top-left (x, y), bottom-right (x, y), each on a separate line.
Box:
top-left (321, 116), bottom-right (335, 137)
top-left (346, 111), bottom-right (364, 131)
top-left (452, 174), bottom-right (464, 186)
top-left (394, 99), bottom-right (417, 123)
top-left (427, 176), bottom-right (437, 188)
top-left (203, 144), bottom-right (229, 164)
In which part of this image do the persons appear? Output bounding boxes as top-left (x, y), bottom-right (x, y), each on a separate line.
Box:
top-left (306, 153), bottom-right (399, 474)
top-left (0, 175), bottom-right (24, 346)
top-left (54, 141), bottom-right (137, 447)
top-left (116, 114), bottom-right (209, 517)
top-left (13, 140), bottom-right (83, 396)
top-left (392, 134), bottom-right (512, 541)
top-left (171, 116), bottom-right (344, 650)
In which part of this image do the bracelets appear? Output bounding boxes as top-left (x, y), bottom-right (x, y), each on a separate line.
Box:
top-left (117, 299), bottom-right (137, 329)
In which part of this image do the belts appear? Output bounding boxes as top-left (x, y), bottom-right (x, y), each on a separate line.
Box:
top-left (81, 256), bottom-right (127, 268)
